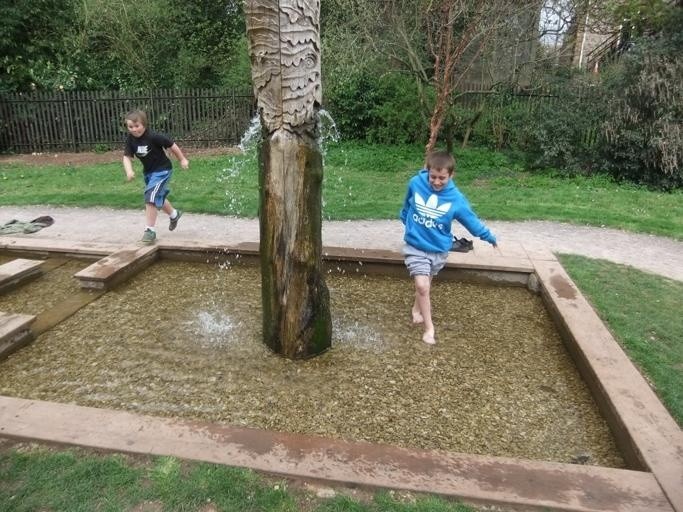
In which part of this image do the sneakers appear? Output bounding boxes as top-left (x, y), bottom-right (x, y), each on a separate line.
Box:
top-left (169, 210), bottom-right (182, 231)
top-left (449, 238), bottom-right (473, 252)
top-left (142, 230), bottom-right (156, 242)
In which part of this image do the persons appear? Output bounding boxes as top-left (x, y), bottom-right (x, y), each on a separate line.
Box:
top-left (122, 110), bottom-right (189, 246)
top-left (401, 152), bottom-right (498, 345)
